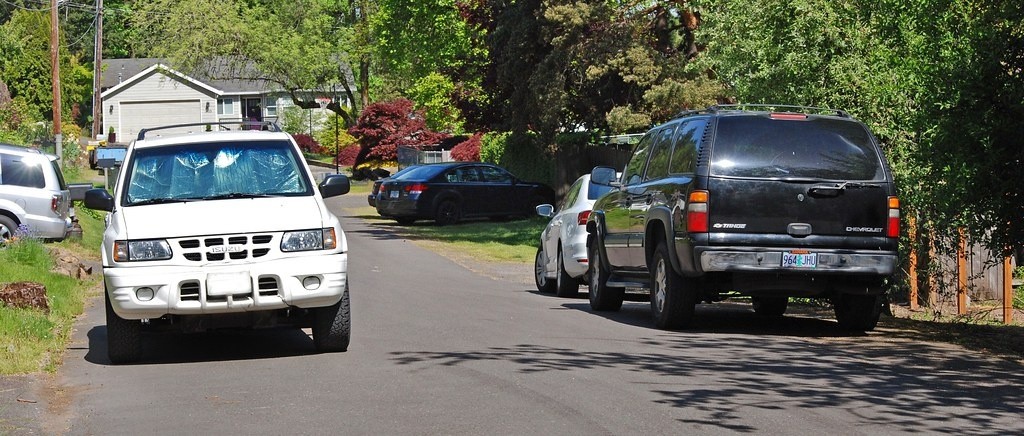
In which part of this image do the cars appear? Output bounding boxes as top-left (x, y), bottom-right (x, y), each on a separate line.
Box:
top-left (368, 161), bottom-right (557, 227)
top-left (534, 171), bottom-right (622, 298)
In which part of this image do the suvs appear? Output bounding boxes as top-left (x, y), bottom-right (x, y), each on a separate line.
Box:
top-left (0, 144), bottom-right (74, 250)
top-left (83, 121), bottom-right (351, 365)
top-left (585, 103), bottom-right (900, 332)
